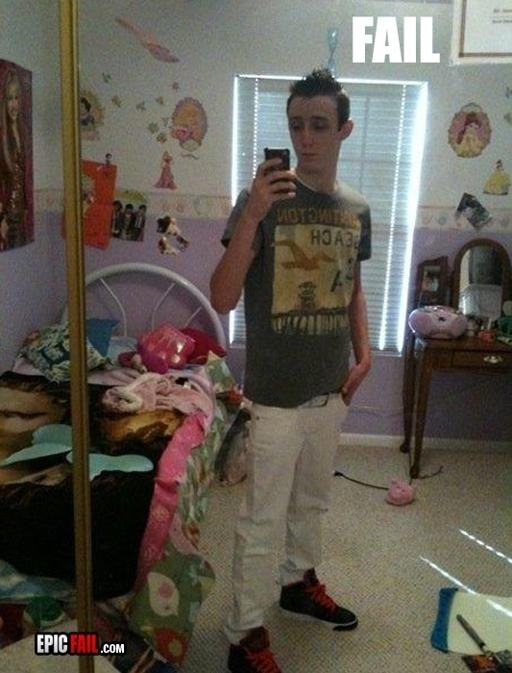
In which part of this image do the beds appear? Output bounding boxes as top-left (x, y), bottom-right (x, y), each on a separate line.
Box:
top-left (0, 257), bottom-right (225, 635)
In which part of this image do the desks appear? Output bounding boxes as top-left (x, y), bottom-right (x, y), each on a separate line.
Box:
top-left (392, 331), bottom-right (512, 478)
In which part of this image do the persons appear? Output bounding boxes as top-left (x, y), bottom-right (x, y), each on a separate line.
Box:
top-left (79, 97), bottom-right (95, 126)
top-left (209, 68), bottom-right (372, 672)
top-left (109, 200), bottom-right (147, 241)
top-left (1, 68), bottom-right (32, 251)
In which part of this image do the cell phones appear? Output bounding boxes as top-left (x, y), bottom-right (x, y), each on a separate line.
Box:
top-left (264, 147), bottom-right (290, 194)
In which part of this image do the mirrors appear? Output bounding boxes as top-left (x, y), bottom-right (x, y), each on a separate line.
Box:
top-left (451, 236), bottom-right (510, 328)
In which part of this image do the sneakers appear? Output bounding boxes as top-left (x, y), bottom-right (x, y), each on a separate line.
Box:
top-left (278, 567), bottom-right (358, 631)
top-left (228, 627), bottom-right (282, 673)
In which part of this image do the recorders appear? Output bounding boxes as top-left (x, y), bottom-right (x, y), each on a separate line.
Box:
top-left (408, 305), bottom-right (467, 339)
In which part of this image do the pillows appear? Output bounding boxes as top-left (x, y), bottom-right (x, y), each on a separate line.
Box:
top-left (178, 325), bottom-right (226, 364)
top-left (17, 317), bottom-right (107, 385)
top-left (136, 321), bottom-right (194, 372)
